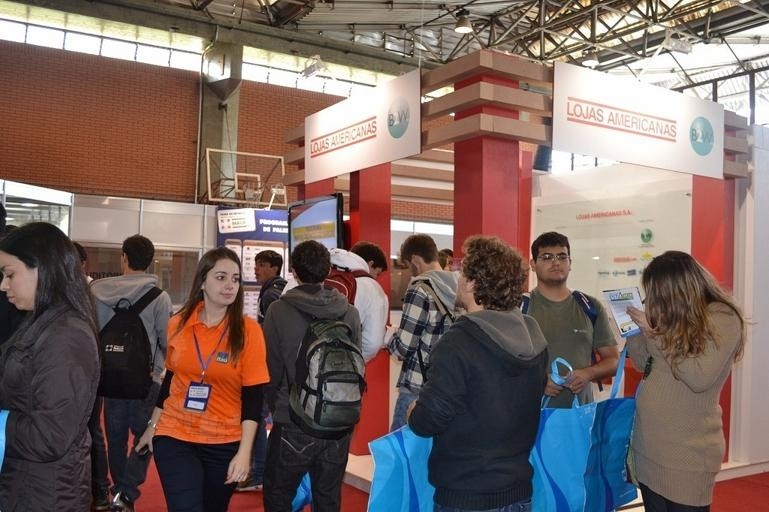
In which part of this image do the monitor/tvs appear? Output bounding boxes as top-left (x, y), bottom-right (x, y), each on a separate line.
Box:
top-left (288, 193), bottom-right (343, 273)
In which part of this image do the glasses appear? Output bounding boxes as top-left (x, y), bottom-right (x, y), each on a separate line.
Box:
top-left (537, 254), bottom-right (569, 263)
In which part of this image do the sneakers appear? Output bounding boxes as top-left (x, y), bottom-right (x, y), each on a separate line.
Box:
top-left (236, 479), bottom-right (263, 492)
top-left (93, 484), bottom-right (134, 512)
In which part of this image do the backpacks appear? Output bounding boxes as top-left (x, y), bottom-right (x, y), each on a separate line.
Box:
top-left (324, 264), bottom-right (372, 304)
top-left (288, 306), bottom-right (365, 440)
top-left (97, 285), bottom-right (162, 402)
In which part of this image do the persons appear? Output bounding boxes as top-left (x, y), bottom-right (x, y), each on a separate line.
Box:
top-left (88, 234), bottom-right (173, 511)
top-left (627, 249), bottom-right (747, 512)
top-left (0, 203), bottom-right (105, 512)
top-left (134, 248), bottom-right (273, 512)
top-left (228, 232), bottom-right (620, 512)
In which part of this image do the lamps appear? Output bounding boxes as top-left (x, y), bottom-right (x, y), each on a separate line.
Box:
top-left (581, 43), bottom-right (600, 68)
top-left (455, 10), bottom-right (473, 37)
